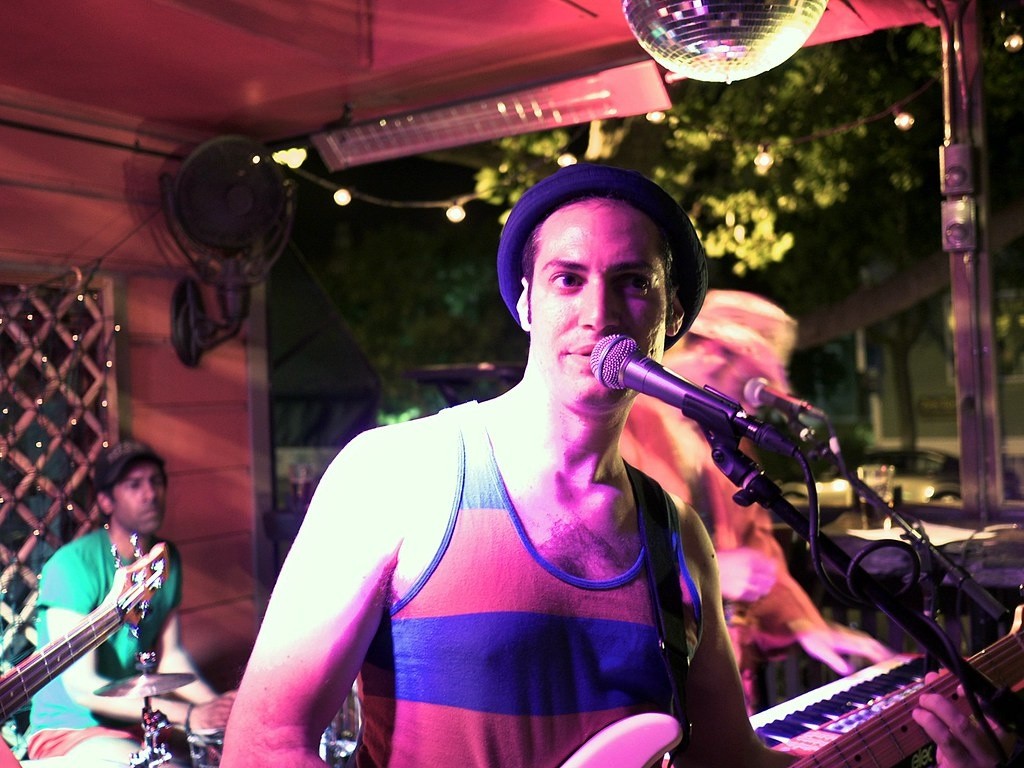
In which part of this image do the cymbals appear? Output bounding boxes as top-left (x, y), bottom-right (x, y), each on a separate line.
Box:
top-left (93, 673), bottom-right (199, 697)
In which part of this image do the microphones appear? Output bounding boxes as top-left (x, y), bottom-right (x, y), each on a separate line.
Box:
top-left (590, 334), bottom-right (796, 458)
top-left (744, 377), bottom-right (822, 419)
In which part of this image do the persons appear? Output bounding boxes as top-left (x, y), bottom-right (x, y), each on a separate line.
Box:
top-left (219, 164), bottom-right (1022, 768)
top-left (616, 289), bottom-right (895, 715)
top-left (24, 442), bottom-right (237, 768)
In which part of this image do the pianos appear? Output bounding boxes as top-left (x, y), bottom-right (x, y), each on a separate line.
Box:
top-left (747, 644), bottom-right (941, 760)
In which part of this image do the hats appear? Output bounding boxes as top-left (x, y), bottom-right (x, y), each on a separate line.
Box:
top-left (497, 162), bottom-right (708, 352)
top-left (691, 288), bottom-right (796, 397)
top-left (95, 440), bottom-right (167, 488)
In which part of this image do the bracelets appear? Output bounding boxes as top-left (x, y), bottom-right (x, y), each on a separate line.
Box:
top-left (184, 703), bottom-right (196, 731)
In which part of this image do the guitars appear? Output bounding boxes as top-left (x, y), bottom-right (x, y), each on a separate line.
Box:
top-left (555, 572), bottom-right (1024, 768)
top-left (0, 528), bottom-right (171, 730)
